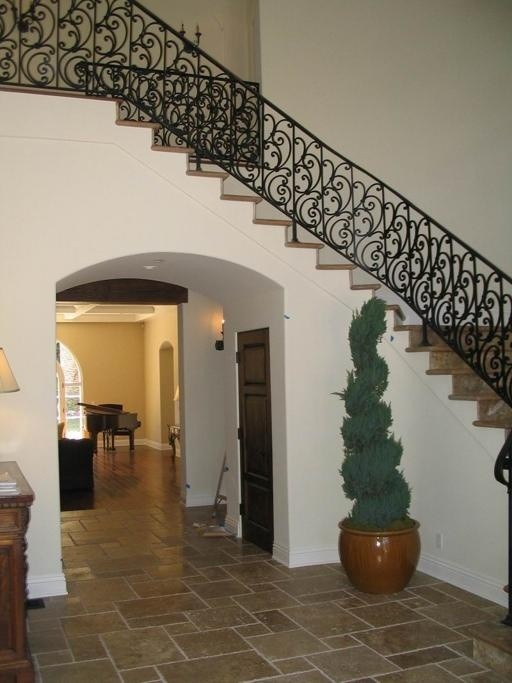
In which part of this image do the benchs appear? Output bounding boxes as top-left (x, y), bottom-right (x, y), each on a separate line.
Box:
top-left (101, 430), bottom-right (129, 452)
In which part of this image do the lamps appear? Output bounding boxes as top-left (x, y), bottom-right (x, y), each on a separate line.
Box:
top-left (0, 346), bottom-right (23, 395)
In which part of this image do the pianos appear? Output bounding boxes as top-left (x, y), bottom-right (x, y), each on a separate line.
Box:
top-left (78, 403), bottom-right (141, 455)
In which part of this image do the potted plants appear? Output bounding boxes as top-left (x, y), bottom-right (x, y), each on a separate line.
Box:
top-left (334, 291), bottom-right (425, 597)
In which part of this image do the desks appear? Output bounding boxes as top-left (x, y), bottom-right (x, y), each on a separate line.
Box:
top-left (165, 422), bottom-right (183, 462)
top-left (1, 458), bottom-right (42, 680)
